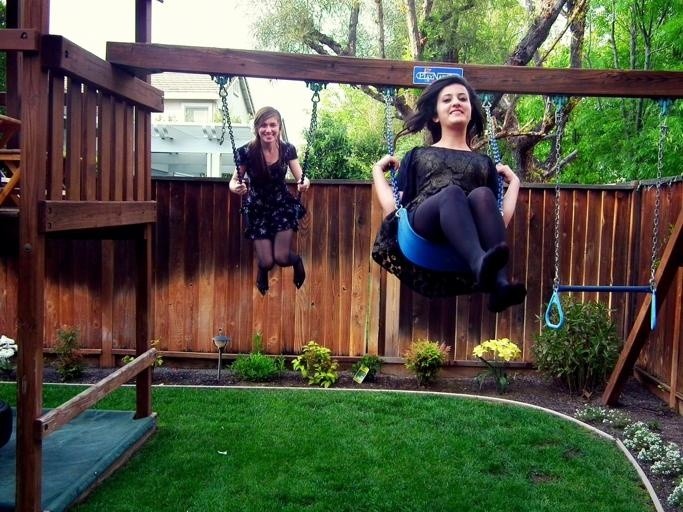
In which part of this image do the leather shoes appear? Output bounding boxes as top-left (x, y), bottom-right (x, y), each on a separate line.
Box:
top-left (256, 267), bottom-right (271, 297)
top-left (293, 257), bottom-right (306, 289)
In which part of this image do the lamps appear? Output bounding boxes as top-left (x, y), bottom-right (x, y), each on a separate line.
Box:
top-left (212, 329), bottom-right (230, 381)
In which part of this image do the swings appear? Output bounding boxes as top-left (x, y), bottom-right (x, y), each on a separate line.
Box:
top-left (545, 96), bottom-right (666, 330)
top-left (385, 88), bottom-right (504, 272)
top-left (219, 86), bottom-right (320, 231)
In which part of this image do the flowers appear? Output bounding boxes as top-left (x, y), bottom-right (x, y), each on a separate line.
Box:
top-left (400, 337), bottom-right (451, 381)
top-left (468, 335), bottom-right (528, 391)
top-left (0, 334), bottom-right (19, 371)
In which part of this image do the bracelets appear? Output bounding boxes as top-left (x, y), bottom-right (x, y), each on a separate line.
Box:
top-left (371, 164), bottom-right (386, 171)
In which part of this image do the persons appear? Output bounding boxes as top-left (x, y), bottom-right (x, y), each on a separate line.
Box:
top-left (371, 74), bottom-right (527, 313)
top-left (227, 108), bottom-right (309, 294)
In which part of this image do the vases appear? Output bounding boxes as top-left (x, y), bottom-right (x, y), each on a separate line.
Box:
top-left (0, 401), bottom-right (13, 447)
top-left (479, 373), bottom-right (501, 388)
